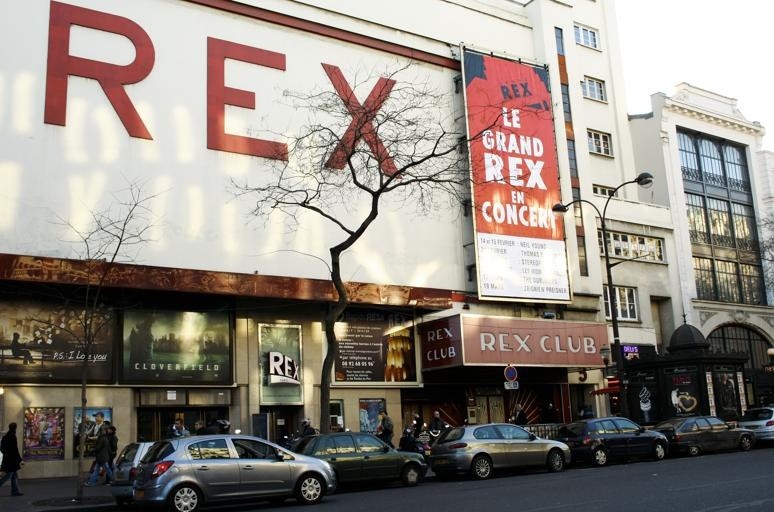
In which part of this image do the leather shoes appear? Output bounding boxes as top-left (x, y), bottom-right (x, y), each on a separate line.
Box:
top-left (12, 492), bottom-right (24, 496)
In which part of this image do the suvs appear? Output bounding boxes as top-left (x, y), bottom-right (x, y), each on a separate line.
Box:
top-left (737, 404), bottom-right (774, 440)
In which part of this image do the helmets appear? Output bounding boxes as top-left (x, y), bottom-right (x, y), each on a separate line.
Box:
top-left (301, 420), bottom-right (309, 426)
top-left (217, 420), bottom-right (231, 432)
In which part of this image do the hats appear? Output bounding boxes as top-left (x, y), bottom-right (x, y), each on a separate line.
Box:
top-left (92, 412), bottom-right (104, 418)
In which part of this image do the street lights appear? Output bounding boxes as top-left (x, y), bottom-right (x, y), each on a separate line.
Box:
top-left (548, 170), bottom-right (654, 414)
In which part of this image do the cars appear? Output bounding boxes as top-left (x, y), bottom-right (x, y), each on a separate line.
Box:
top-left (559, 416), bottom-right (668, 466)
top-left (296, 433), bottom-right (427, 485)
top-left (430, 424), bottom-right (571, 478)
top-left (135, 436), bottom-right (336, 511)
top-left (108, 440), bottom-right (153, 501)
top-left (649, 416), bottom-right (754, 455)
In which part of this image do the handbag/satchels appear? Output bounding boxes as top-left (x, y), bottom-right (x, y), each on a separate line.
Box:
top-left (90, 461), bottom-right (105, 476)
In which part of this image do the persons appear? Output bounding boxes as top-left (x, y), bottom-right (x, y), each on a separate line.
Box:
top-left (216, 419), bottom-right (232, 434)
top-left (301, 418), bottom-right (316, 436)
top-left (168, 418), bottom-right (192, 439)
top-left (82, 419), bottom-right (119, 488)
top-left (194, 419), bottom-right (210, 436)
top-left (12, 332), bottom-right (34, 366)
top-left (74, 409), bottom-right (108, 459)
top-left (398, 410), bottom-right (447, 466)
top-left (511, 403), bottom-right (528, 426)
top-left (378, 410), bottom-right (396, 449)
top-left (719, 372), bottom-right (737, 410)
top-left (0, 422), bottom-right (25, 496)
top-left (537, 399), bottom-right (558, 424)
